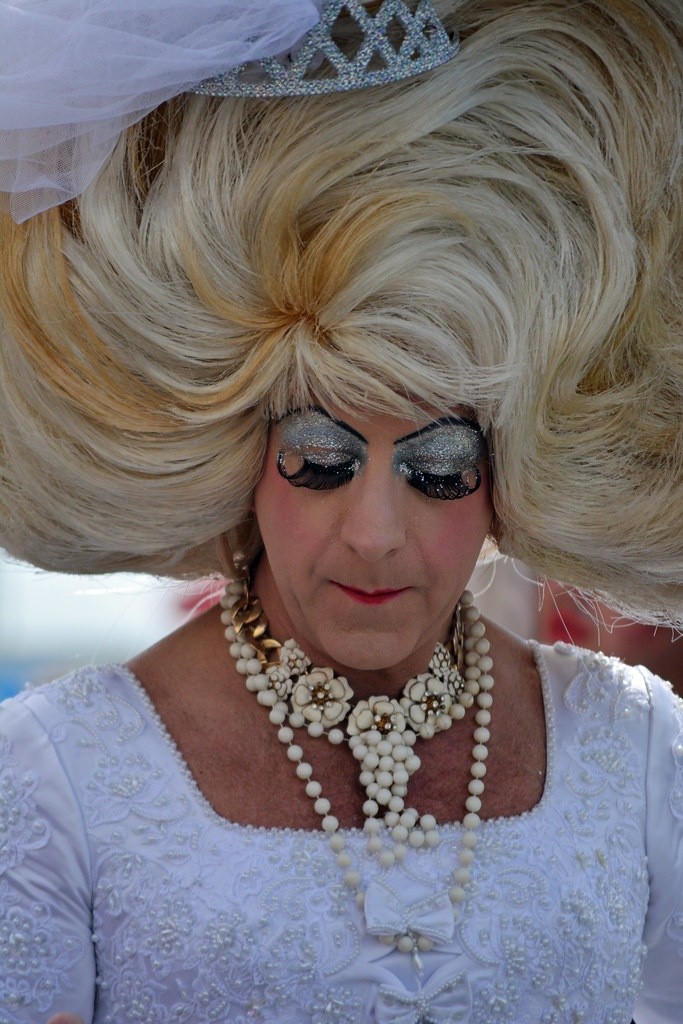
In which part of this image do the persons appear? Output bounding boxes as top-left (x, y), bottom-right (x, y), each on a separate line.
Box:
top-left (0, 0), bottom-right (683, 1024)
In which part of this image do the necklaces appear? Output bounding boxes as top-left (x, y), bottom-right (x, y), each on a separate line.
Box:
top-left (220, 582), bottom-right (494, 952)
